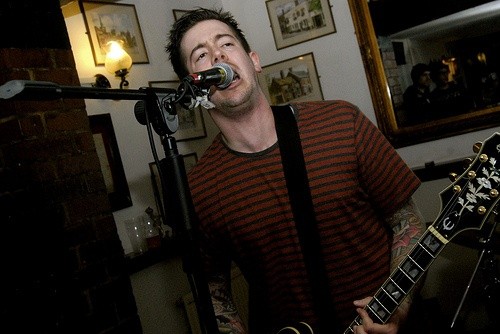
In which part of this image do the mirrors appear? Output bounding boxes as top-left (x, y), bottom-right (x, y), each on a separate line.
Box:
top-left (346, 0), bottom-right (500, 150)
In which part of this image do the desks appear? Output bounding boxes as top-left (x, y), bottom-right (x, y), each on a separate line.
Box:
top-left (122, 231), bottom-right (220, 334)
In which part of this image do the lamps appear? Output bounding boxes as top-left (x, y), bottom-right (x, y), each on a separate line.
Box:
top-left (103, 38), bottom-right (132, 90)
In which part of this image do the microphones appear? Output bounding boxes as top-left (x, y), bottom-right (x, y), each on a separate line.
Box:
top-left (185, 62), bottom-right (234, 88)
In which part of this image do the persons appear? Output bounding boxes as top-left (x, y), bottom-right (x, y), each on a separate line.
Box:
top-left (169, 7), bottom-right (428, 334)
top-left (400, 59), bottom-right (500, 139)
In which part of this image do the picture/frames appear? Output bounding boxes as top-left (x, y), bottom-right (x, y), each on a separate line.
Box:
top-left (87, 113), bottom-right (135, 213)
top-left (147, 152), bottom-right (200, 230)
top-left (78, 0), bottom-right (151, 67)
top-left (257, 52), bottom-right (325, 106)
top-left (148, 79), bottom-right (208, 145)
top-left (264, 0), bottom-right (338, 52)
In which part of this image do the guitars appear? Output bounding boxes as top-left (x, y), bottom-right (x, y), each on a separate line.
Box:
top-left (277, 133), bottom-right (500, 334)
